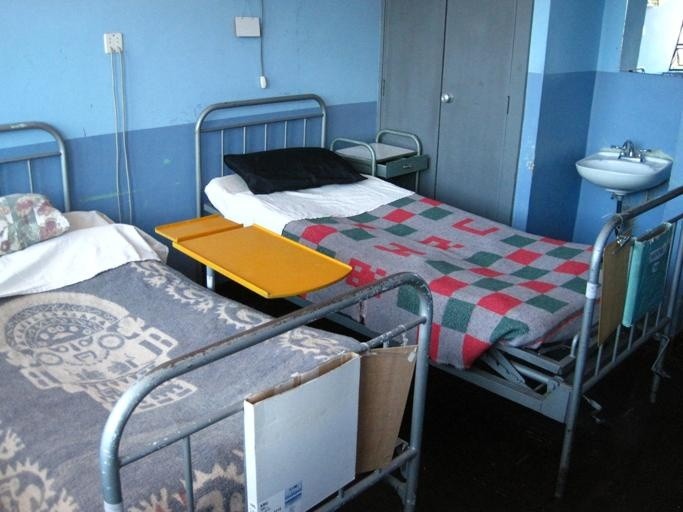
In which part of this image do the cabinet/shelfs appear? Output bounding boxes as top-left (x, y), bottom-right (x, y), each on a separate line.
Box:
top-left (375, 0), bottom-right (537, 229)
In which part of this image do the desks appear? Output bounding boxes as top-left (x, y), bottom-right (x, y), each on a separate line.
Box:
top-left (152, 212), bottom-right (353, 302)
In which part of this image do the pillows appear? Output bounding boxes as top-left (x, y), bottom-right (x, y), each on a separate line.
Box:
top-left (0, 192), bottom-right (71, 257)
top-left (217, 142), bottom-right (369, 195)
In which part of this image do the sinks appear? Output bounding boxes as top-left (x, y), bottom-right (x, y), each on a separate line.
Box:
top-left (582, 160), bottom-right (655, 190)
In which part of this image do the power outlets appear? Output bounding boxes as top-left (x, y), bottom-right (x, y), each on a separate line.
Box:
top-left (102, 32), bottom-right (123, 55)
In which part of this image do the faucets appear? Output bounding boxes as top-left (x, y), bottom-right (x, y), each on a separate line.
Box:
top-left (622, 139), bottom-right (636, 157)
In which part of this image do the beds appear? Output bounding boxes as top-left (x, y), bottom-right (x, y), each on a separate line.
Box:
top-left (0, 117), bottom-right (433, 510)
top-left (187, 90), bottom-right (681, 504)
